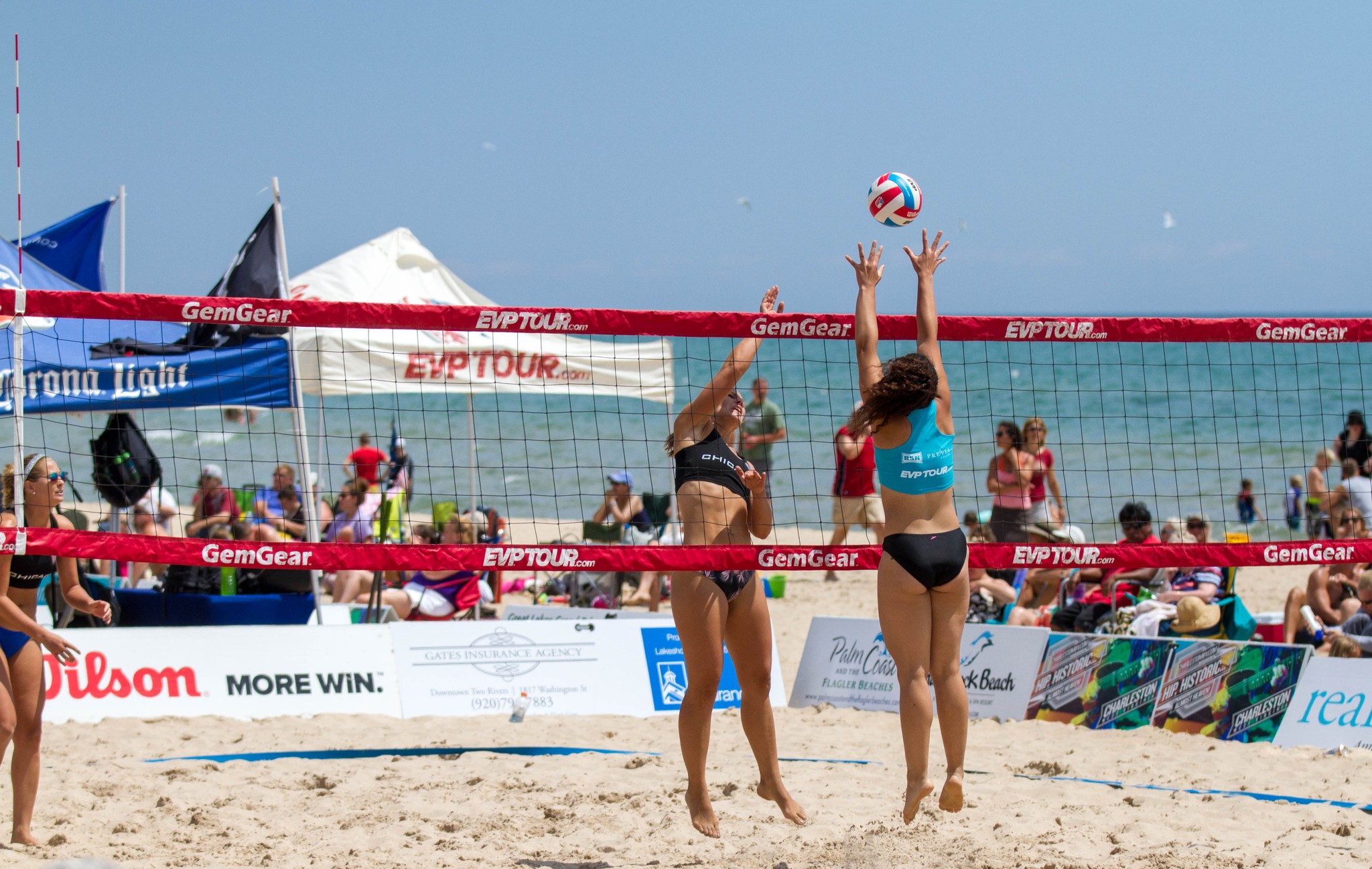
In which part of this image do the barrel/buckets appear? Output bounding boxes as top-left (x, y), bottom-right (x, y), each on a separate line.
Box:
top-left (768, 574), bottom-right (786, 598)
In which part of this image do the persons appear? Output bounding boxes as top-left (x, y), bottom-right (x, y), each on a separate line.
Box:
top-left (0, 229), bottom-right (1371, 840)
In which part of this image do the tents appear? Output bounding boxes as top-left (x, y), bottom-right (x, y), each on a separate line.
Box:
top-left (0, 228), bottom-right (684, 593)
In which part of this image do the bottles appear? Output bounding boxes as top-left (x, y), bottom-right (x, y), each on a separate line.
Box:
top-left (1300, 605), bottom-right (1324, 641)
top-left (547, 596), bottom-right (569, 604)
top-left (220, 567), bottom-right (237, 597)
top-left (1161, 579), bottom-right (1171, 594)
top-left (536, 593), bottom-right (547, 603)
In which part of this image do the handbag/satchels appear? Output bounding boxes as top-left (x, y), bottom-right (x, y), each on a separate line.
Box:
top-left (1225, 589), bottom-right (1257, 642)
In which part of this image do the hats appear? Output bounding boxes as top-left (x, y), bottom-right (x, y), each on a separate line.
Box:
top-left (1346, 411), bottom-right (1364, 428)
top-left (1051, 526), bottom-right (1086, 545)
top-left (1171, 596), bottom-right (1221, 630)
top-left (1021, 519), bottom-right (1059, 544)
top-left (607, 472), bottom-right (633, 487)
top-left (201, 465), bottom-right (223, 481)
top-left (1186, 511), bottom-right (1210, 525)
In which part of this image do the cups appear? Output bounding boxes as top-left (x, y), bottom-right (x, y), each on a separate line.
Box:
top-left (1136, 585), bottom-right (1150, 603)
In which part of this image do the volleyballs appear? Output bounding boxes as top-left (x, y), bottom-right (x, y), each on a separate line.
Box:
top-left (866, 172), bottom-right (922, 228)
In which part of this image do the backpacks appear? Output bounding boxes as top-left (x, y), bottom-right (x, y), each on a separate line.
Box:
top-left (89, 412), bottom-right (164, 508)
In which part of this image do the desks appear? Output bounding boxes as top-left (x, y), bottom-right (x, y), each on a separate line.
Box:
top-left (111, 587), bottom-right (316, 627)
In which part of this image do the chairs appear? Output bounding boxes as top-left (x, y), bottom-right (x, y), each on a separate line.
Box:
top-left (225, 483), bottom-right (678, 627)
top-left (44, 581), bottom-right (98, 629)
top-left (985, 565), bottom-right (1241, 640)
top-left (62, 510), bottom-right (88, 531)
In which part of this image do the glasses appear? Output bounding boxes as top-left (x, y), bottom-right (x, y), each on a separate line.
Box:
top-left (1187, 522), bottom-right (1206, 530)
top-left (1162, 529), bottom-right (1171, 534)
top-left (996, 430), bottom-right (1006, 438)
top-left (1343, 516), bottom-right (1358, 525)
top-left (1026, 427), bottom-right (1043, 432)
top-left (1359, 598), bottom-right (1372, 605)
top-left (49, 471), bottom-right (68, 484)
top-left (341, 493), bottom-right (350, 499)
